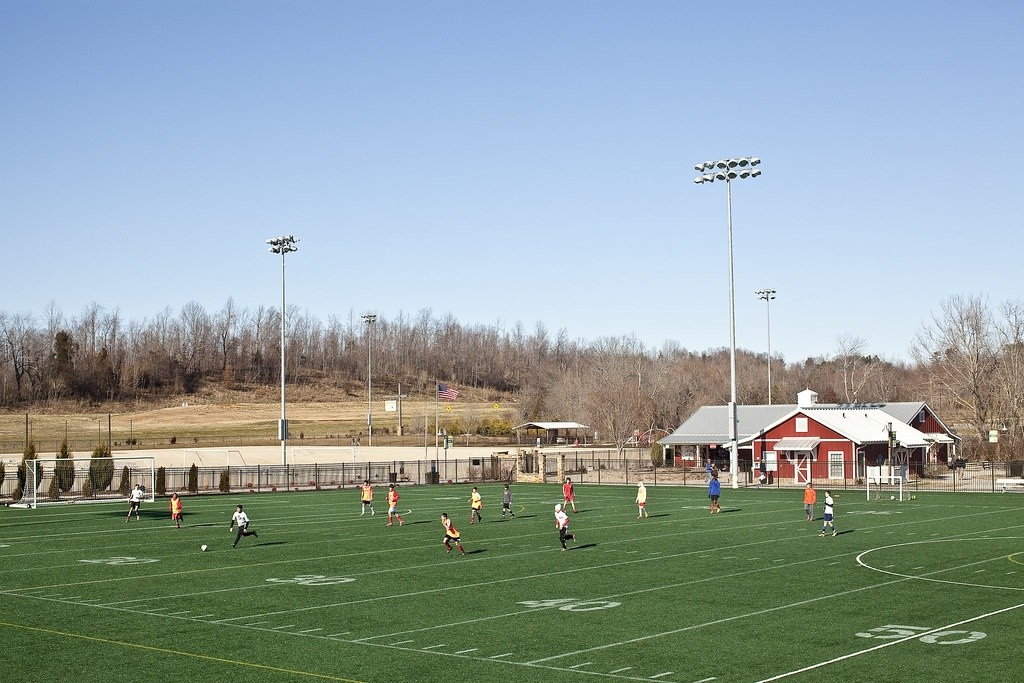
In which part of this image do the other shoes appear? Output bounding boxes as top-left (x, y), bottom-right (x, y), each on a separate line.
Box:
top-left (399, 522), bottom-right (403, 526)
top-left (387, 524), bottom-right (393, 527)
top-left (446, 548), bottom-right (452, 554)
top-left (573, 509), bottom-right (578, 513)
top-left (560, 549), bottom-right (568, 552)
top-left (252, 531), bottom-right (258, 537)
top-left (832, 530), bottom-right (837, 537)
top-left (818, 534), bottom-right (826, 538)
top-left (715, 509), bottom-right (720, 513)
top-left (808, 517), bottom-right (813, 522)
top-left (231, 543), bottom-right (235, 548)
top-left (572, 535), bottom-right (576, 542)
top-left (710, 510), bottom-right (715, 513)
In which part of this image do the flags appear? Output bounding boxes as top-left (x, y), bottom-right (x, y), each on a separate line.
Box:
top-left (438, 383), bottom-right (459, 400)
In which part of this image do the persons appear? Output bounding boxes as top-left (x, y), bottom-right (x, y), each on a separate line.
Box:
top-left (360, 480), bottom-right (376, 516)
top-left (636, 482), bottom-right (648, 519)
top-left (169, 493), bottom-right (183, 529)
top-left (126, 483), bottom-right (143, 522)
top-left (467, 487), bottom-right (482, 524)
top-left (955, 455), bottom-right (965, 480)
top-left (229, 505), bottom-right (258, 547)
top-left (562, 477), bottom-right (579, 512)
top-left (818, 491), bottom-right (838, 537)
top-left (555, 504), bottom-right (577, 551)
top-left (385, 484), bottom-right (404, 526)
top-left (758, 459), bottom-right (768, 485)
top-left (705, 458), bottom-right (721, 514)
top-left (441, 513), bottom-right (465, 555)
top-left (501, 484), bottom-right (515, 519)
top-left (804, 483), bottom-right (817, 521)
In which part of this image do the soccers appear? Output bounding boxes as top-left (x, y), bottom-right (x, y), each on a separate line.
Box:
top-left (201, 544), bottom-right (208, 551)
